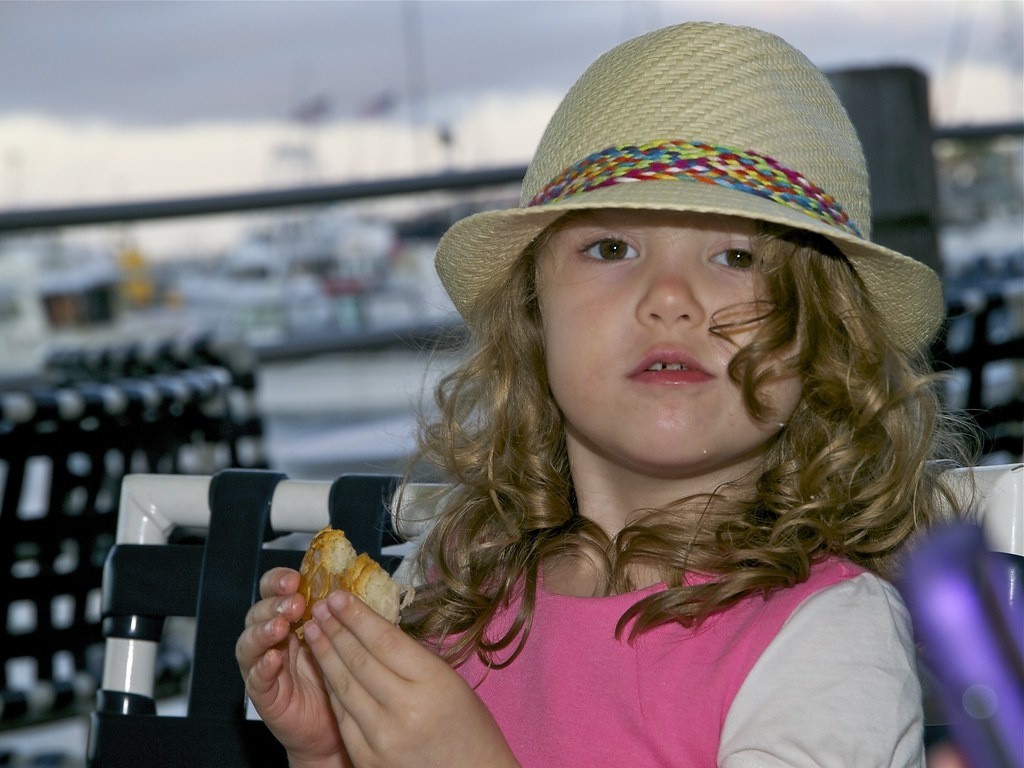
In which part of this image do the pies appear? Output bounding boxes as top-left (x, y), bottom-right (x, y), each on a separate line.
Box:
top-left (292, 526), bottom-right (400, 650)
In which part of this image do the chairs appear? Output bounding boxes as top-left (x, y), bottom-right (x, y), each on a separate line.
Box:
top-left (88, 462), bottom-right (1024, 768)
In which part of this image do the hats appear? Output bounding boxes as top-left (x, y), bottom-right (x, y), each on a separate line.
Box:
top-left (432, 20), bottom-right (946, 365)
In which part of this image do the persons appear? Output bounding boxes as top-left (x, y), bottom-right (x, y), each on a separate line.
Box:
top-left (237, 26), bottom-right (946, 768)
